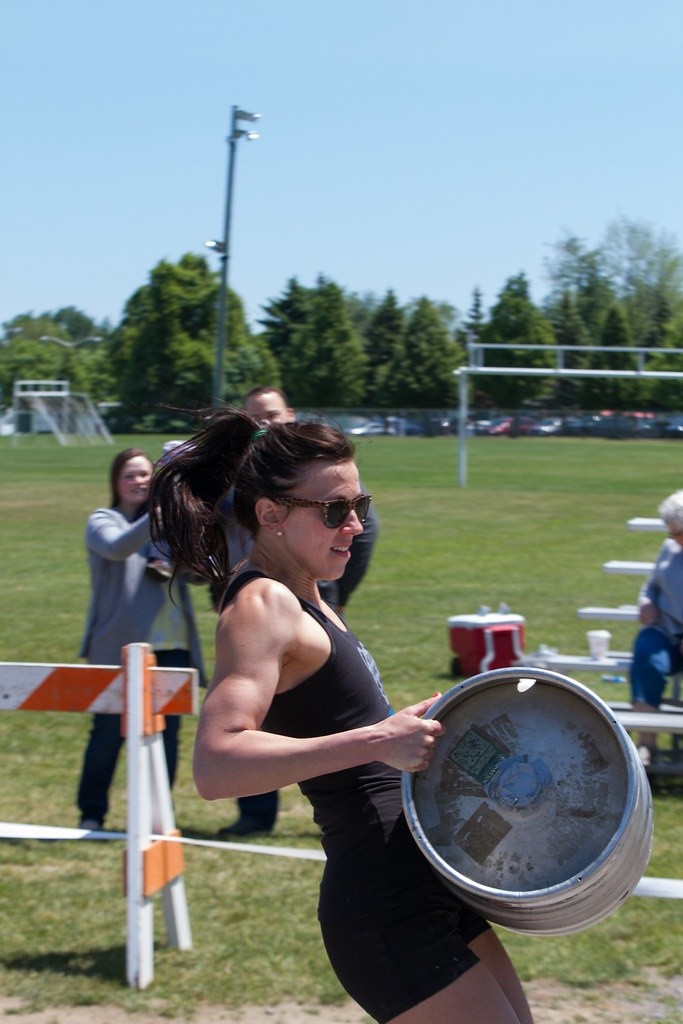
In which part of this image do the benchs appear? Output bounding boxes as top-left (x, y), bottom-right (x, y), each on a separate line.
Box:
top-left (524, 516), bottom-right (683, 780)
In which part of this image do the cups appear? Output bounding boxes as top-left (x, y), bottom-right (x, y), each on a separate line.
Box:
top-left (586, 630), bottom-right (612, 661)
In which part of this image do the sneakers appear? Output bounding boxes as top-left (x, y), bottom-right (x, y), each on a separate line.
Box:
top-left (637, 742), bottom-right (659, 766)
top-left (217, 816), bottom-right (273, 841)
top-left (78, 820), bottom-right (104, 832)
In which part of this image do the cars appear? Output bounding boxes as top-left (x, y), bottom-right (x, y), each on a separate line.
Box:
top-left (289, 409), bottom-right (683, 443)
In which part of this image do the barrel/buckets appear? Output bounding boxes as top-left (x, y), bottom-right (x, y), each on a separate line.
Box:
top-left (401, 665), bottom-right (657, 935)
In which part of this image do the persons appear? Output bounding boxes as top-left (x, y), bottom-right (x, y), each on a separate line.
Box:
top-left (142, 404), bottom-right (533, 1023)
top-left (70, 447), bottom-right (209, 835)
top-left (618, 489), bottom-right (683, 768)
top-left (216, 386), bottom-right (377, 840)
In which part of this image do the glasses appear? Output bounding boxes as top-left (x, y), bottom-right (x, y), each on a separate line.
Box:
top-left (271, 494), bottom-right (373, 529)
